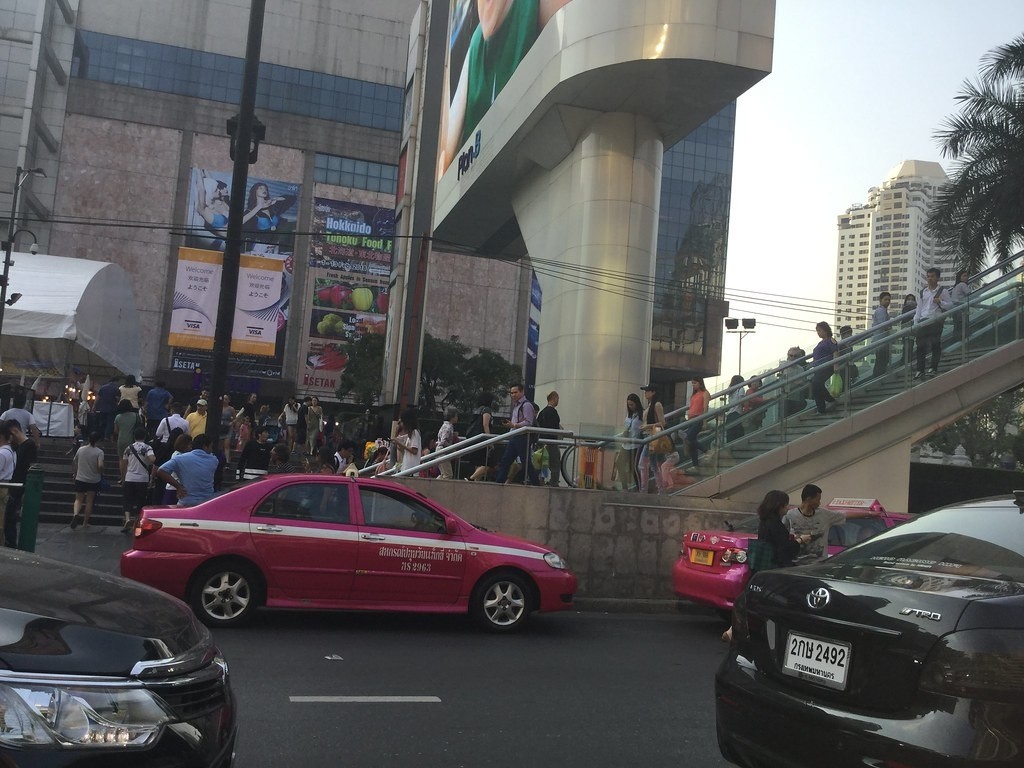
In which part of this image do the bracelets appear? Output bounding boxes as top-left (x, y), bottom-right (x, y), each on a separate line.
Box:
top-left (869, 511), bottom-right (872, 517)
top-left (799, 537), bottom-right (803, 544)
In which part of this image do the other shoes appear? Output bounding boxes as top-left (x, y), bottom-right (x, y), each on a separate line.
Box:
top-left (812, 412), bottom-right (823, 416)
top-left (464, 476), bottom-right (475, 481)
top-left (71, 515), bottom-right (80, 528)
top-left (704, 451), bottom-right (715, 464)
top-left (121, 520), bottom-right (133, 532)
top-left (686, 467), bottom-right (700, 473)
top-left (722, 632), bottom-right (731, 641)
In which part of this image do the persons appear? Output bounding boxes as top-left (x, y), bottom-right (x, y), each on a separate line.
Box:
top-left (779, 322), bottom-right (859, 417)
top-left (873, 292), bottom-right (892, 378)
top-left (686, 376), bottom-right (714, 472)
top-left (857, 527), bottom-right (879, 542)
top-left (914, 269), bottom-right (952, 380)
top-left (753, 490), bottom-right (811, 575)
top-left (71, 375), bottom-right (219, 533)
top-left (220, 392), bottom-right (355, 481)
top-left (365, 384), bottom-right (563, 486)
top-left (902, 294), bottom-right (916, 362)
top-left (782, 484), bottom-right (882, 567)
top-left (195, 170), bottom-right (297, 255)
top-left (0, 393), bottom-right (41, 548)
top-left (954, 270), bottom-right (971, 343)
top-left (442, 0), bottom-right (570, 171)
top-left (617, 385), bottom-right (681, 496)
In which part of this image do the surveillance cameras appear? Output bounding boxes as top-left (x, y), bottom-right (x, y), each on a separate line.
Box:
top-left (29, 243), bottom-right (39, 255)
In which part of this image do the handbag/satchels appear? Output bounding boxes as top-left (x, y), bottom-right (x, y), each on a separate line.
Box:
top-left (319, 415), bottom-right (323, 432)
top-left (101, 475), bottom-right (110, 491)
top-left (375, 463), bottom-right (390, 477)
top-left (623, 430), bottom-right (629, 436)
top-left (533, 444), bottom-right (549, 469)
top-left (650, 422), bottom-right (672, 451)
top-left (827, 371), bottom-right (843, 399)
top-left (466, 407), bottom-right (493, 439)
top-left (219, 424), bottom-right (229, 435)
top-left (747, 523), bottom-right (780, 570)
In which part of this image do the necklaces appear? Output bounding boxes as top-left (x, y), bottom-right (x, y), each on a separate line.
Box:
top-left (726, 375), bottom-right (778, 460)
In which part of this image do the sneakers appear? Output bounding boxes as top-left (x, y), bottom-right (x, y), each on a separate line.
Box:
top-left (913, 369), bottom-right (925, 381)
top-left (926, 368), bottom-right (936, 376)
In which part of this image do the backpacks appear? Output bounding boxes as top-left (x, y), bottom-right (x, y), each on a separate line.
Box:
top-left (521, 398), bottom-right (541, 443)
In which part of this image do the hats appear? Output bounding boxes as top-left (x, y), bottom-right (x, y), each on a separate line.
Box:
top-left (196, 399), bottom-right (207, 405)
top-left (640, 382), bottom-right (659, 391)
top-left (219, 395), bottom-right (230, 402)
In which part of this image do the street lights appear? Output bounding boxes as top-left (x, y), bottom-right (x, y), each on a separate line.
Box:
top-left (0, 167), bottom-right (48, 337)
top-left (724, 318), bottom-right (757, 376)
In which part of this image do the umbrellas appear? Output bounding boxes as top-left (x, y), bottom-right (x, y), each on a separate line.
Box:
top-left (79, 375), bottom-right (90, 427)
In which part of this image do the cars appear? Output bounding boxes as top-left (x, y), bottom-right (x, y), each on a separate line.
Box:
top-left (0, 546), bottom-right (239, 768)
top-left (119, 461), bottom-right (576, 634)
top-left (671, 498), bottom-right (919, 628)
top-left (714, 489), bottom-right (1024, 768)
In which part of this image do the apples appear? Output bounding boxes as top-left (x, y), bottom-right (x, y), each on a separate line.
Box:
top-left (317, 284), bottom-right (387, 313)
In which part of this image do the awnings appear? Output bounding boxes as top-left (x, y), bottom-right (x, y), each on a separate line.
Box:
top-left (1, 254), bottom-right (140, 381)
